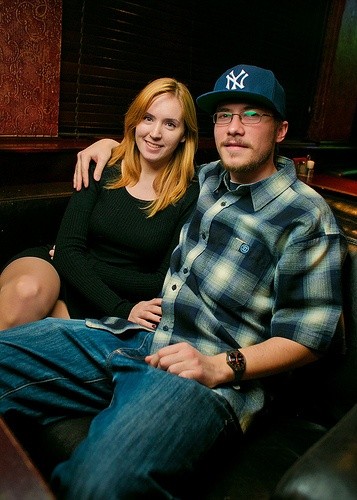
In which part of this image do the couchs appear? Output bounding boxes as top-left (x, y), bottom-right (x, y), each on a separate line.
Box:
top-left (26, 197), bottom-right (357, 500)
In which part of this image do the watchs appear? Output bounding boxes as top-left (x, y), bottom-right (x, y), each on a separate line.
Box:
top-left (226, 350), bottom-right (246, 390)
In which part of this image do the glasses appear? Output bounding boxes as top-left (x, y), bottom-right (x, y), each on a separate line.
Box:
top-left (210, 111), bottom-right (274, 128)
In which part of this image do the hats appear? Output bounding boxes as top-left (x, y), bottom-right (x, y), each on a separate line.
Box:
top-left (195, 65), bottom-right (290, 122)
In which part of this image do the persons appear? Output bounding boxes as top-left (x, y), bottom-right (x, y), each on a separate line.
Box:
top-left (0, 64), bottom-right (342, 500)
